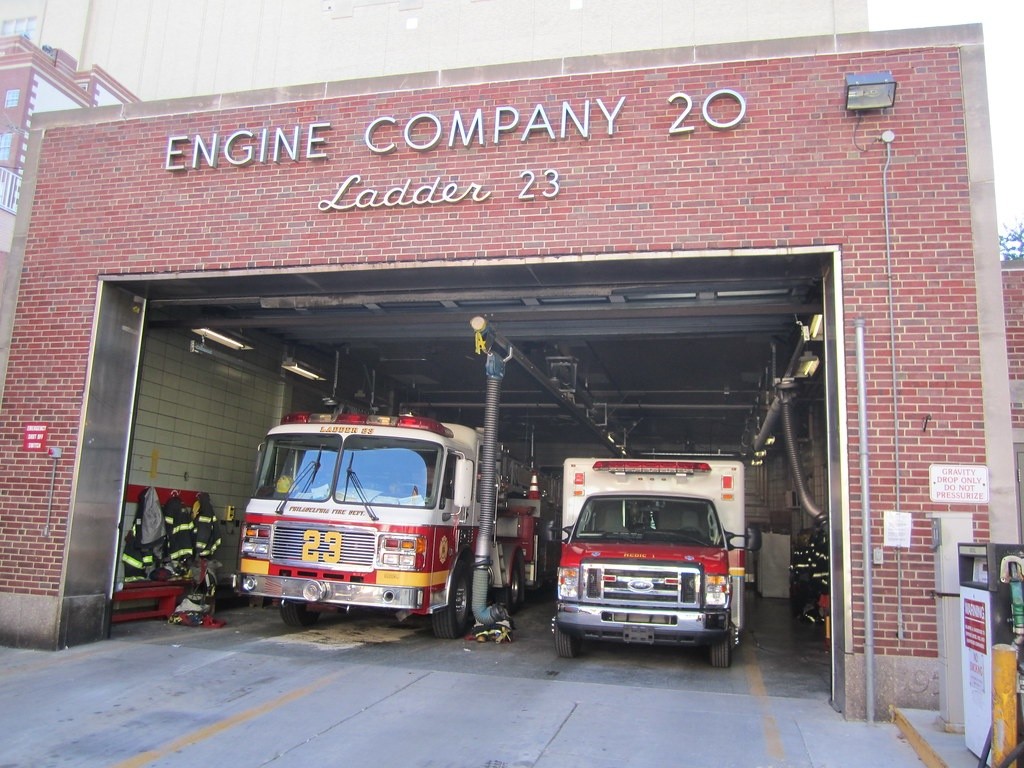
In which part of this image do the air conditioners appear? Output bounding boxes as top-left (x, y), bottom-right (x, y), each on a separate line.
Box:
top-left (784, 490), bottom-right (800, 509)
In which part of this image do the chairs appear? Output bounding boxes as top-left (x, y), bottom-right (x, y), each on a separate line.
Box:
top-left (677, 511), bottom-right (705, 536)
top-left (597, 510), bottom-right (629, 533)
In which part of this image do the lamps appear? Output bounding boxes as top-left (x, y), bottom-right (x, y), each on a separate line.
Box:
top-left (755, 449), bottom-right (767, 457)
top-left (765, 434), bottom-right (776, 445)
top-left (808, 313), bottom-right (824, 341)
top-left (751, 459), bottom-right (763, 466)
top-left (324, 399), bottom-right (339, 406)
top-left (398, 408), bottom-right (418, 417)
top-left (793, 351), bottom-right (820, 378)
top-left (845, 71), bottom-right (896, 111)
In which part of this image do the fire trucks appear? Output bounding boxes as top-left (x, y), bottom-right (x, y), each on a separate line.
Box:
top-left (230, 411), bottom-right (563, 639)
top-left (540, 456), bottom-right (763, 670)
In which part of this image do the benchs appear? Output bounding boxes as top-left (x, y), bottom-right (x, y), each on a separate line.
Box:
top-left (281, 356), bottom-right (328, 381)
top-left (191, 326), bottom-right (255, 351)
top-left (112, 580), bottom-right (212, 622)
